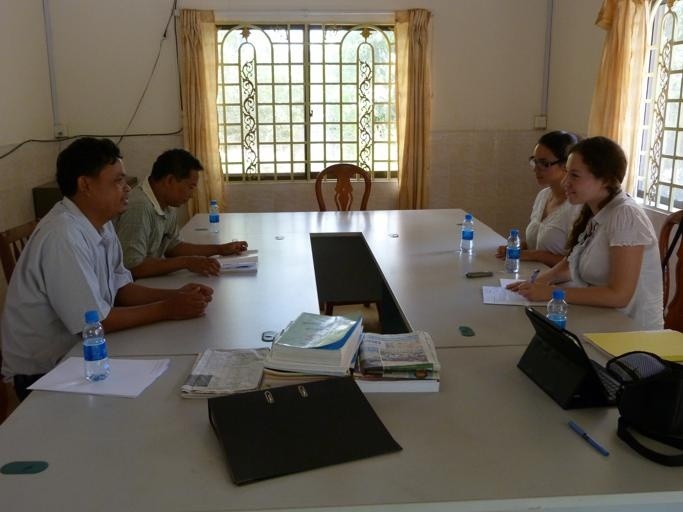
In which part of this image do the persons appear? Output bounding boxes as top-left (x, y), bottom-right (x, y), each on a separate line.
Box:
top-left (494, 130), bottom-right (589, 268)
top-left (506, 136), bottom-right (665, 332)
top-left (115, 148), bottom-right (248, 279)
top-left (1, 139), bottom-right (217, 404)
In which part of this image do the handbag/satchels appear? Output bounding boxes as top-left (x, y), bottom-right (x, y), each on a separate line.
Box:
top-left (605, 350), bottom-right (682, 467)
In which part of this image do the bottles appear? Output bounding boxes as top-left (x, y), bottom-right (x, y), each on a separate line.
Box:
top-left (82, 310), bottom-right (111, 381)
top-left (460, 213), bottom-right (474, 258)
top-left (547, 289), bottom-right (568, 330)
top-left (505, 229), bottom-right (521, 272)
top-left (209, 199), bottom-right (220, 234)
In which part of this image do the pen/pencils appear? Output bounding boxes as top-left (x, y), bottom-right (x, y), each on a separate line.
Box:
top-left (531, 270), bottom-right (540, 283)
top-left (568, 421), bottom-right (609, 456)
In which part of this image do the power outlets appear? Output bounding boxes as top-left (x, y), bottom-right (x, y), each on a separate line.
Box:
top-left (53, 125), bottom-right (68, 139)
top-left (533, 116), bottom-right (548, 129)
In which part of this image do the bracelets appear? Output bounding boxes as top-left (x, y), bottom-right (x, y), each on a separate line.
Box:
top-left (545, 285), bottom-right (555, 301)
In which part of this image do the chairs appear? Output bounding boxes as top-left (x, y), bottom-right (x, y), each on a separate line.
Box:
top-left (658, 209), bottom-right (683, 334)
top-left (0, 219), bottom-right (39, 288)
top-left (315, 163), bottom-right (371, 317)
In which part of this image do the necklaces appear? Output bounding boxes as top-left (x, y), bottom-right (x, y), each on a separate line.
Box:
top-left (549, 193), bottom-right (564, 210)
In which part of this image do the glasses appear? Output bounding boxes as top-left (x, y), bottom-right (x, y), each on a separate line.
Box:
top-left (528, 156), bottom-right (562, 168)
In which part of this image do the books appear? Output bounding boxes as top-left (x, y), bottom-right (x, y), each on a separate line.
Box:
top-left (579, 327), bottom-right (683, 364)
top-left (179, 310), bottom-right (440, 400)
top-left (481, 279), bottom-right (569, 310)
top-left (209, 248), bottom-right (259, 273)
top-left (25, 354), bottom-right (169, 398)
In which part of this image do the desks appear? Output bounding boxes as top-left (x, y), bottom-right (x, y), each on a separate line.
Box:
top-left (0, 207), bottom-right (683, 511)
top-left (32, 176), bottom-right (139, 220)
